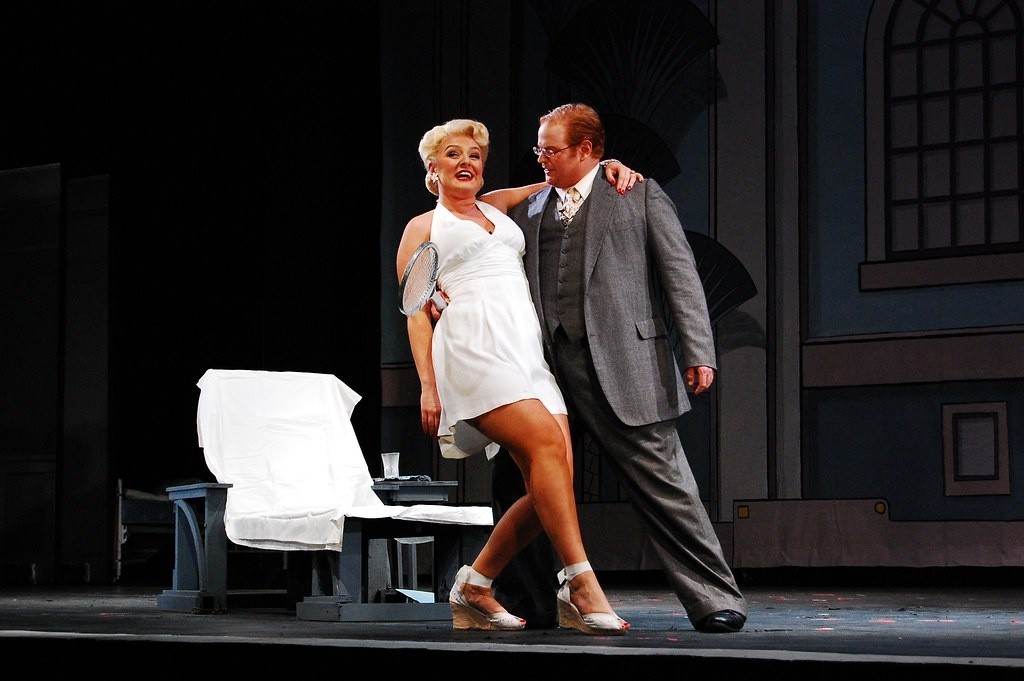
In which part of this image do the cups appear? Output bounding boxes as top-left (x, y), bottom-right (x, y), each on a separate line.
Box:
top-left (381, 453), bottom-right (399, 480)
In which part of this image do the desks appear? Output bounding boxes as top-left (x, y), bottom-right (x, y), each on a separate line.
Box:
top-left (374, 479), bottom-right (459, 592)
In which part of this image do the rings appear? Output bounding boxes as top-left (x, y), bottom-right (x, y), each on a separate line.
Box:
top-left (630, 169), bottom-right (635, 174)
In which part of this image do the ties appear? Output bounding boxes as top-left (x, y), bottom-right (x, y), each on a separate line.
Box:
top-left (559, 188), bottom-right (581, 227)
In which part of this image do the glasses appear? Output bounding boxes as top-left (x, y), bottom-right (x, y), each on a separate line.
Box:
top-left (533, 143), bottom-right (581, 158)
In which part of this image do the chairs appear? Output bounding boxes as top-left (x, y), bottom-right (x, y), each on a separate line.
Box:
top-left (157, 368), bottom-right (494, 623)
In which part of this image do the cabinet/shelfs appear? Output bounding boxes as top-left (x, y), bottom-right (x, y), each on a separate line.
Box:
top-left (114, 478), bottom-right (175, 584)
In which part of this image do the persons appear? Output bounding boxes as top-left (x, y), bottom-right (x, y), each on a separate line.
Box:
top-left (430, 95), bottom-right (748, 635)
top-left (396, 118), bottom-right (643, 635)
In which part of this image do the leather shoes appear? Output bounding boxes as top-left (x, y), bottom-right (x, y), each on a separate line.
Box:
top-left (706, 609), bottom-right (744, 632)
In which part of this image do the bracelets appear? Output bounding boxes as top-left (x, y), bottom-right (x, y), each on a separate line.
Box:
top-left (599, 158), bottom-right (621, 168)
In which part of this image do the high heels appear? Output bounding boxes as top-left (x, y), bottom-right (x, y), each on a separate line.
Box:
top-left (556, 560), bottom-right (629, 636)
top-left (448, 564), bottom-right (527, 631)
